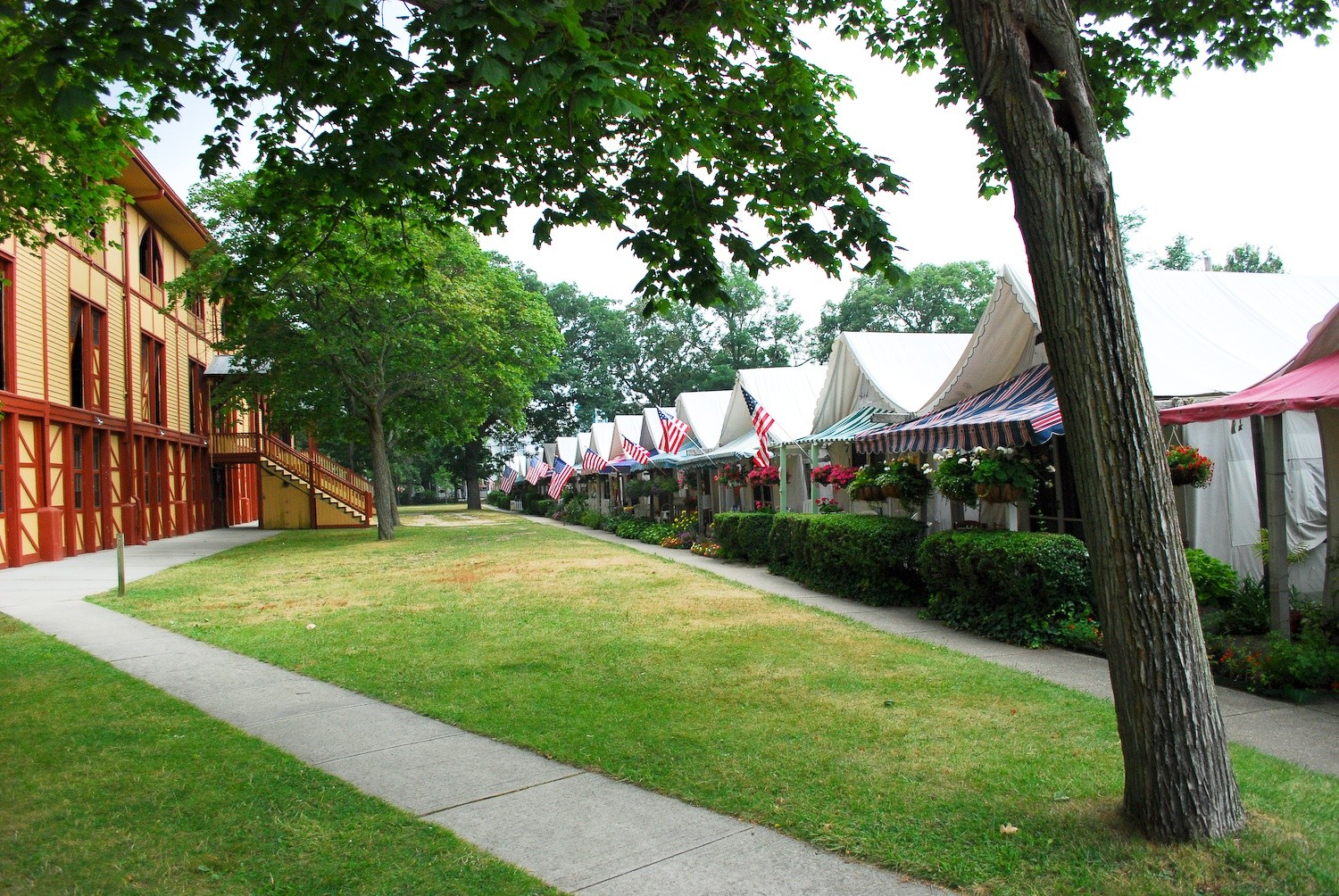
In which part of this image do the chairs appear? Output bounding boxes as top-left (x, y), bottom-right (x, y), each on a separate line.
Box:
top-left (654, 504), bottom-right (669, 523)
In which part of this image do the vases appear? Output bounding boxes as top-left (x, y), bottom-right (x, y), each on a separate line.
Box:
top-left (858, 487), bottom-right (880, 501)
top-left (975, 481), bottom-right (1023, 503)
top-left (1290, 611), bottom-right (1303, 632)
top-left (882, 485), bottom-right (915, 499)
top-left (728, 480), bottom-right (743, 486)
top-left (1170, 468), bottom-right (1194, 486)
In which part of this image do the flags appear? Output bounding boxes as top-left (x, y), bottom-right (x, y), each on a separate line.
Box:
top-left (525, 455), bottom-right (548, 486)
top-left (741, 385), bottom-right (774, 469)
top-left (658, 408), bottom-right (690, 455)
top-left (548, 457), bottom-right (574, 500)
top-left (623, 437), bottom-right (650, 464)
top-left (500, 465), bottom-right (518, 494)
top-left (583, 448), bottom-right (607, 471)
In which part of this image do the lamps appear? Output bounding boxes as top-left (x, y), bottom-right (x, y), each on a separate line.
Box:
top-left (159, 430), bottom-right (165, 437)
top-left (96, 417), bottom-right (103, 425)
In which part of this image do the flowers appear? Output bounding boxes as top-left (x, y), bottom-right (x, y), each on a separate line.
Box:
top-left (921, 445), bottom-right (1058, 510)
top-left (846, 464), bottom-right (878, 502)
top-left (714, 464), bottom-right (745, 493)
top-left (877, 455), bottom-right (933, 511)
top-left (746, 466), bottom-right (791, 487)
top-left (678, 470), bottom-right (689, 486)
top-left (1166, 444), bottom-right (1217, 487)
top-left (809, 465), bottom-right (857, 489)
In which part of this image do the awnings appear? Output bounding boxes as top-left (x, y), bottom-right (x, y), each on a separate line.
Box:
top-left (854, 363), bottom-right (1065, 454)
top-left (1156, 349), bottom-right (1339, 427)
top-left (776, 407), bottom-right (895, 446)
top-left (572, 427), bottom-right (773, 475)
